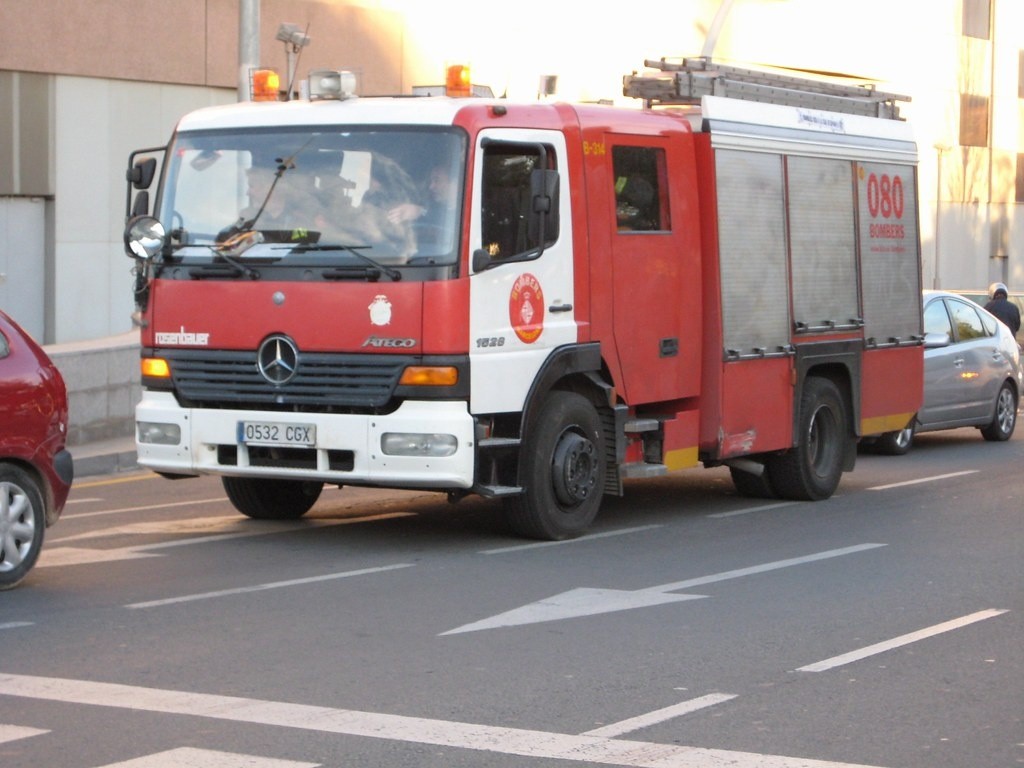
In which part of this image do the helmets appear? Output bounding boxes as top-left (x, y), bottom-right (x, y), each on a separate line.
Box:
top-left (988, 282), bottom-right (1008, 301)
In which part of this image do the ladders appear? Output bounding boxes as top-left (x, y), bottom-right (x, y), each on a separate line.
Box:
top-left (621, 56), bottom-right (913, 121)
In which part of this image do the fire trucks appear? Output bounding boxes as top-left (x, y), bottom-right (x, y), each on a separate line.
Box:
top-left (122, 56), bottom-right (926, 543)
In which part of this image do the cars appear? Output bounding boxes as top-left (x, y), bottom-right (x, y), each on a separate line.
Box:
top-left (0, 308), bottom-right (75, 591)
top-left (856, 288), bottom-right (1022, 455)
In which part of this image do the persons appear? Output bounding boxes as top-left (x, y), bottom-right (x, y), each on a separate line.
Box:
top-left (222, 158), bottom-right (656, 266)
top-left (985, 283), bottom-right (1020, 336)
